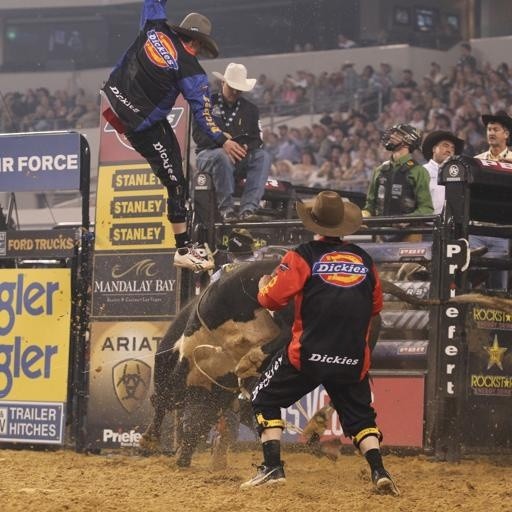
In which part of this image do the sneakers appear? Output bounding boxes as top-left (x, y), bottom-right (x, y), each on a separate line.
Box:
top-left (221, 210), bottom-right (240, 223)
top-left (241, 461), bottom-right (287, 489)
top-left (371, 467), bottom-right (402, 497)
top-left (243, 210), bottom-right (260, 222)
top-left (173, 241), bottom-right (208, 269)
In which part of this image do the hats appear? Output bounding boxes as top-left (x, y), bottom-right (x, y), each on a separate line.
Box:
top-left (482, 111), bottom-right (512, 134)
top-left (211, 63), bottom-right (256, 92)
top-left (215, 227), bottom-right (267, 255)
top-left (423, 130), bottom-right (465, 160)
top-left (295, 189), bottom-right (362, 237)
top-left (166, 13), bottom-right (219, 58)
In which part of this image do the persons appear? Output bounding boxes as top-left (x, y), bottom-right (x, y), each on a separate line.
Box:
top-left (189, 61), bottom-right (275, 257)
top-left (0, 87), bottom-right (87, 133)
top-left (238, 187), bottom-right (401, 497)
top-left (210, 38), bottom-right (511, 186)
top-left (419, 129), bottom-right (465, 211)
top-left (357, 118), bottom-right (436, 245)
top-left (98, 2), bottom-right (249, 272)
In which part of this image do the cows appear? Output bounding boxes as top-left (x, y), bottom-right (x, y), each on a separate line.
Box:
top-left (136, 245), bottom-right (512, 470)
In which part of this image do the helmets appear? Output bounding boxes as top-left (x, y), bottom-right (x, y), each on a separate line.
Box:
top-left (384, 123), bottom-right (423, 152)
